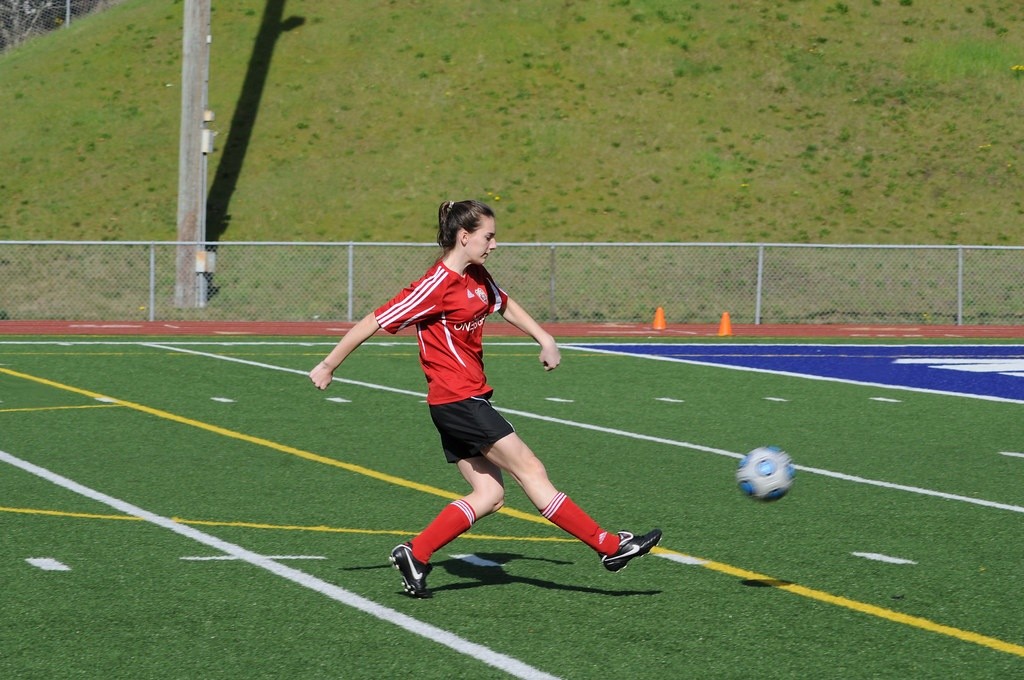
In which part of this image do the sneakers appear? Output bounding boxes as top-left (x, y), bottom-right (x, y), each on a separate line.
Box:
top-left (599, 529), bottom-right (662, 574)
top-left (389, 541), bottom-right (433, 598)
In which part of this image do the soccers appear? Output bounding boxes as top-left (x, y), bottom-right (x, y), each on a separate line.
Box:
top-left (736, 446), bottom-right (796, 503)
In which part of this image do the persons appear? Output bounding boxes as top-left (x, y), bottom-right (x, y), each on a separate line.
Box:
top-left (308, 200), bottom-right (665, 601)
